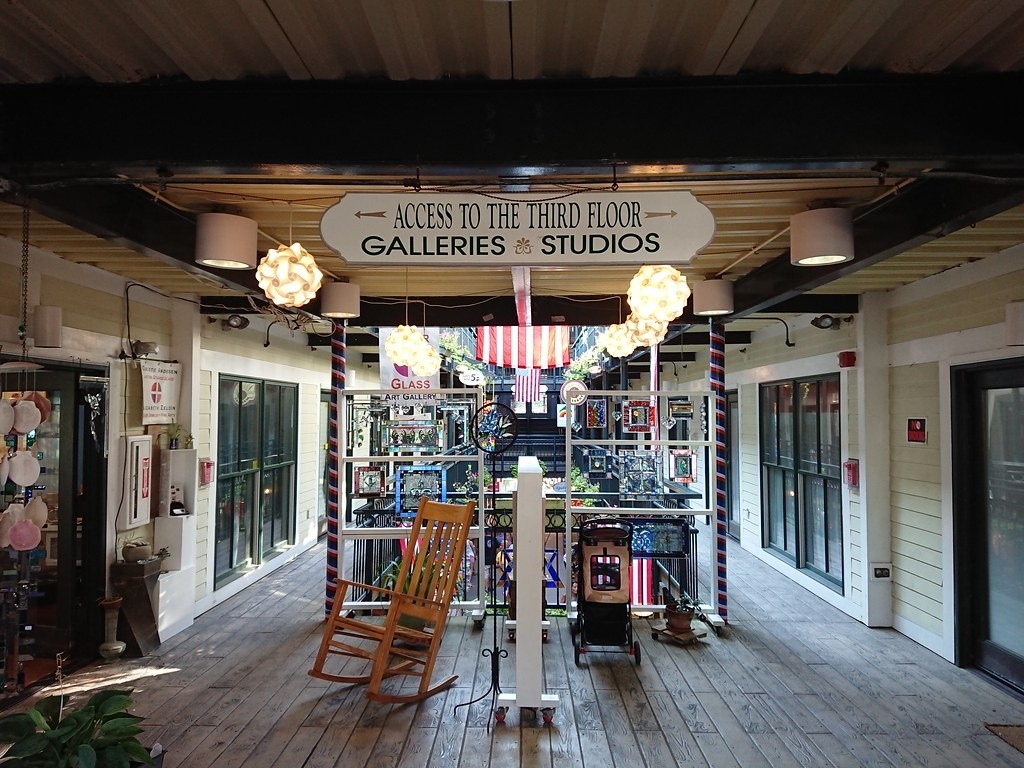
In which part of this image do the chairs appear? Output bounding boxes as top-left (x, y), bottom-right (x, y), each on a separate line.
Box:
top-left (308, 495), bottom-right (478, 704)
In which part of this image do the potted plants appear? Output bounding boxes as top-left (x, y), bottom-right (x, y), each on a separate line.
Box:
top-left (563, 340), bottom-right (608, 380)
top-left (377, 550), bottom-right (463, 633)
top-left (183, 432), bottom-right (195, 449)
top-left (439, 328), bottom-right (495, 381)
top-left (154, 418), bottom-right (187, 449)
top-left (664, 592), bottom-right (702, 634)
top-left (117, 530), bottom-right (152, 562)
top-left (95, 579), bottom-right (128, 658)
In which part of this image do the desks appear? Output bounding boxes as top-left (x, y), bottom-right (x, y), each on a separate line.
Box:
top-left (40, 516), bottom-right (83, 568)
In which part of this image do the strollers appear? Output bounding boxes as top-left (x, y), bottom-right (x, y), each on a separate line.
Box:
top-left (571, 516), bottom-right (643, 668)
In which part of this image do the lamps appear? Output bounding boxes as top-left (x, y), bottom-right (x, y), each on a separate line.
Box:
top-left (384, 266), bottom-right (429, 367)
top-left (626, 265), bottom-right (691, 323)
top-left (790, 199), bottom-right (856, 267)
top-left (255, 201), bottom-right (324, 309)
top-left (693, 273), bottom-right (734, 316)
top-left (602, 323), bottom-right (637, 358)
top-left (625, 309), bottom-right (669, 346)
top-left (32, 305), bottom-right (62, 348)
top-left (195, 205), bottom-right (256, 270)
top-left (410, 304), bottom-right (442, 376)
top-left (321, 275), bottom-right (360, 317)
